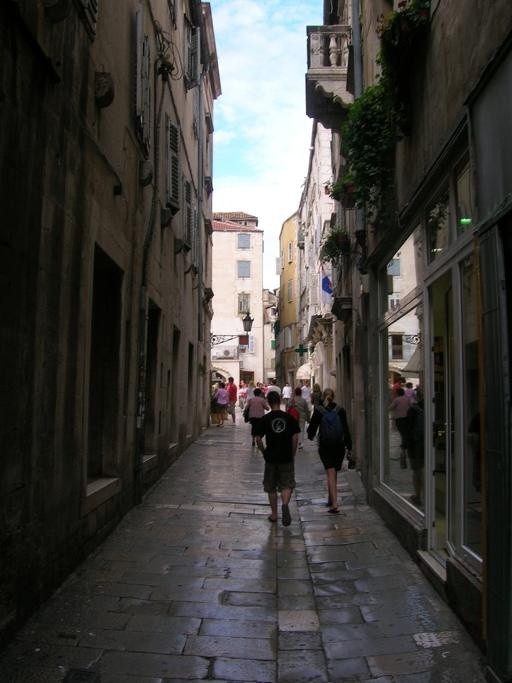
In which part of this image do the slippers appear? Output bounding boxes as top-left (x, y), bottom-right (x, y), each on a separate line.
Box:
top-left (282, 503), bottom-right (291, 526)
top-left (329, 507), bottom-right (339, 513)
top-left (269, 514), bottom-right (277, 523)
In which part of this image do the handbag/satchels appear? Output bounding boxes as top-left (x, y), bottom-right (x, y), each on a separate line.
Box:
top-left (243, 408), bottom-right (249, 422)
top-left (288, 406), bottom-right (299, 422)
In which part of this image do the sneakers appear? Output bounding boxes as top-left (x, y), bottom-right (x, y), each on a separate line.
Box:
top-left (298, 444), bottom-right (303, 449)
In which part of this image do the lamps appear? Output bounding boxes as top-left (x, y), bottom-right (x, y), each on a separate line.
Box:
top-left (210, 310), bottom-right (255, 350)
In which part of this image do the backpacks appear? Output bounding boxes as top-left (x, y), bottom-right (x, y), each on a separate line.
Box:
top-left (317, 404), bottom-right (343, 444)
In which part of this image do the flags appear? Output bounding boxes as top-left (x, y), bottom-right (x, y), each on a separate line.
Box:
top-left (322, 266), bottom-right (333, 305)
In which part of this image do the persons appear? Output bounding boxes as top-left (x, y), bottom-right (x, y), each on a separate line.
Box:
top-left (254, 391), bottom-right (302, 527)
top-left (387, 377), bottom-right (425, 506)
top-left (306, 388), bottom-right (353, 512)
top-left (212, 377), bottom-right (325, 449)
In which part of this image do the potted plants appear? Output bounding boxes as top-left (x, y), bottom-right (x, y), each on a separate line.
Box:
top-left (327, 173), bottom-right (356, 208)
top-left (316, 225), bottom-right (351, 263)
